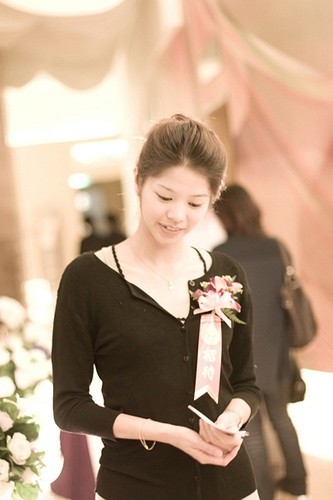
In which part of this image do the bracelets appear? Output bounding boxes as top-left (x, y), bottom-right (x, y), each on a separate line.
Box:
top-left (139, 418), bottom-right (156, 451)
top-left (224, 408), bottom-right (243, 429)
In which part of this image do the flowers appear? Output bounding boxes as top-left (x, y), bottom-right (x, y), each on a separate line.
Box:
top-left (0, 295), bottom-right (52, 500)
top-left (189, 275), bottom-right (248, 328)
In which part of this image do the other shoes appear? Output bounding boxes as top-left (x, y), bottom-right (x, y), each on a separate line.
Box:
top-left (279, 478), bottom-right (305, 495)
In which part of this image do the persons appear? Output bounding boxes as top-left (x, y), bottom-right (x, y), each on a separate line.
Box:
top-left (79, 213), bottom-right (127, 256)
top-left (51, 114), bottom-right (262, 500)
top-left (211, 184), bottom-right (308, 500)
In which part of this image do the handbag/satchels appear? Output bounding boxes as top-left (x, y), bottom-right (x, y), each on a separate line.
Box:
top-left (287, 350), bottom-right (306, 403)
top-left (275, 239), bottom-right (317, 348)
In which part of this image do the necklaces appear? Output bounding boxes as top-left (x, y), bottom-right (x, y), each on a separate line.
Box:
top-left (129, 238), bottom-right (192, 292)
